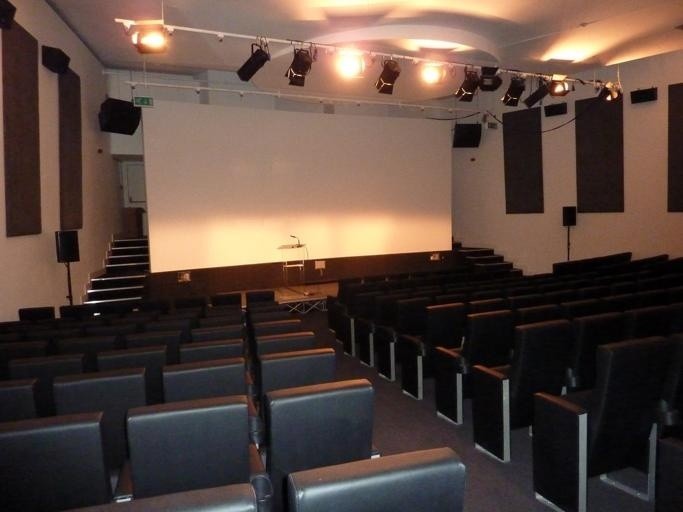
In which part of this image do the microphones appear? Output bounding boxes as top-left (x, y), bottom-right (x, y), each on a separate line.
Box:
top-left (289, 234), bottom-right (300, 245)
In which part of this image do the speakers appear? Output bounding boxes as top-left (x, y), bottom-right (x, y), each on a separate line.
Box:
top-left (562, 205), bottom-right (576, 227)
top-left (630, 87), bottom-right (657, 103)
top-left (0, 1), bottom-right (16, 30)
top-left (41, 45), bottom-right (71, 74)
top-left (98, 98), bottom-right (142, 135)
top-left (544, 103), bottom-right (568, 117)
top-left (55, 230), bottom-right (80, 263)
top-left (452, 124), bottom-right (480, 149)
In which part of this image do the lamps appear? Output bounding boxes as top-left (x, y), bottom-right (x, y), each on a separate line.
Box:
top-left (422, 64), bottom-right (457, 85)
top-left (454, 69), bottom-right (478, 102)
top-left (526, 80), bottom-right (548, 107)
top-left (374, 59), bottom-right (400, 93)
top-left (128, 19), bottom-right (169, 53)
top-left (547, 73), bottom-right (572, 96)
top-left (282, 46), bottom-right (313, 85)
top-left (499, 75), bottom-right (526, 108)
top-left (593, 80), bottom-right (620, 105)
top-left (236, 42), bottom-right (271, 82)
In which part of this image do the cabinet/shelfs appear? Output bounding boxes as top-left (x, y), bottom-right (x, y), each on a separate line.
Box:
top-left (120, 161), bottom-right (146, 208)
